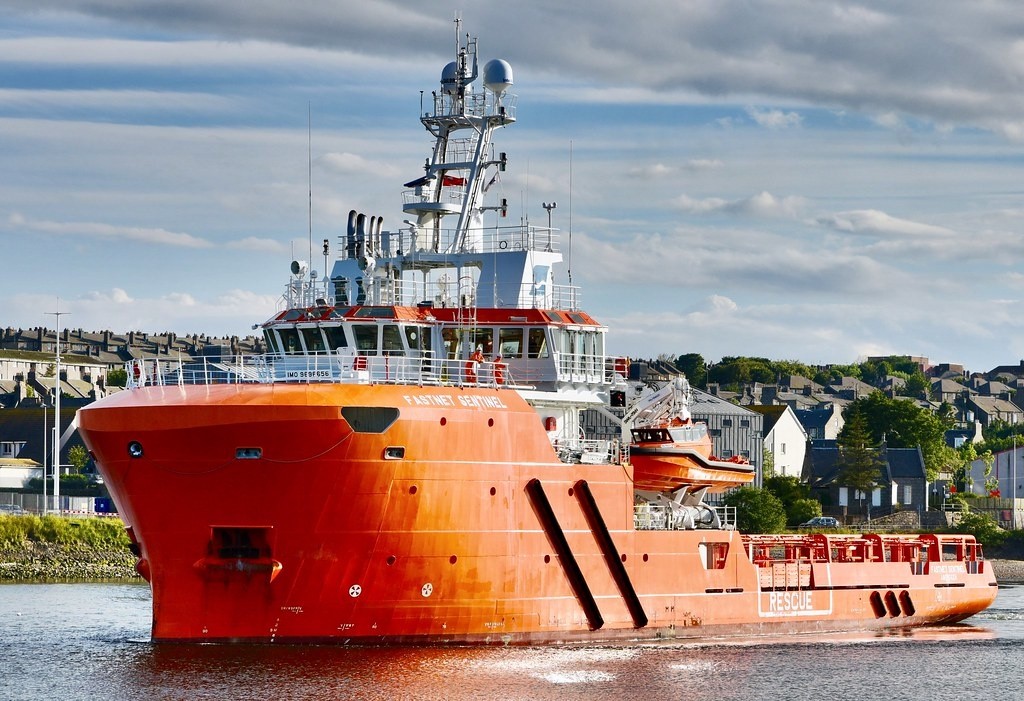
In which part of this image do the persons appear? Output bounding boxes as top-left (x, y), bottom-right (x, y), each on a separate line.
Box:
top-left (465, 347), bottom-right (484, 388)
top-left (493, 353), bottom-right (506, 384)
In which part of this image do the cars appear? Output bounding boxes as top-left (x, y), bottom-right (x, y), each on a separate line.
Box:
top-left (0, 503), bottom-right (21, 515)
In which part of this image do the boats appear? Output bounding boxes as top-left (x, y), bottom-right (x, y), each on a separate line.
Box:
top-left (74, 5), bottom-right (998, 656)
top-left (620, 413), bottom-right (754, 497)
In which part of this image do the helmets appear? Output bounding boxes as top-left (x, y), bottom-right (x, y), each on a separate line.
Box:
top-left (497, 353), bottom-right (502, 358)
top-left (476, 348), bottom-right (482, 351)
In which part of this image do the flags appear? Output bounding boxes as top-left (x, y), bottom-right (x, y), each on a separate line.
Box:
top-left (443, 174), bottom-right (467, 186)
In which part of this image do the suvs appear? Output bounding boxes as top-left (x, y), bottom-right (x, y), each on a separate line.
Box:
top-left (797, 517), bottom-right (839, 531)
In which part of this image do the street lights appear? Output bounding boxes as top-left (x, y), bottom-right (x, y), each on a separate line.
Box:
top-left (1010, 435), bottom-right (1017, 530)
top-left (38, 403), bottom-right (46, 511)
